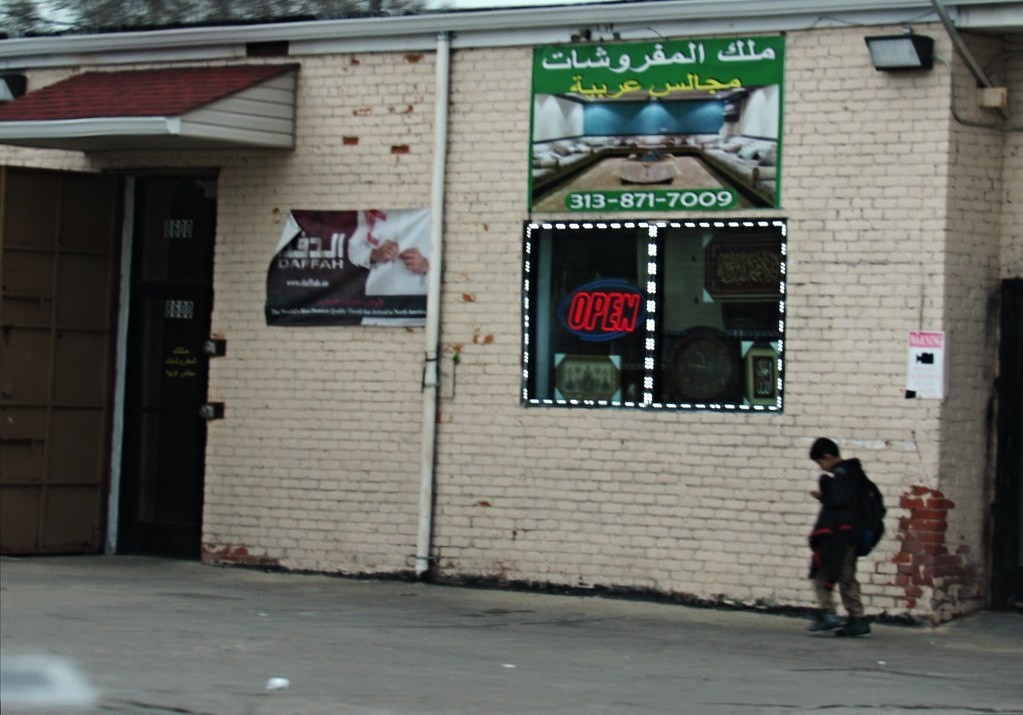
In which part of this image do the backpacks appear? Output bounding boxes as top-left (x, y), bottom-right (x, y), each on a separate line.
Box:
top-left (847, 467), bottom-right (886, 556)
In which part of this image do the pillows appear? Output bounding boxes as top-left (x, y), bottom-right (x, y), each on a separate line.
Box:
top-left (724, 142), bottom-right (741, 153)
top-left (762, 150), bottom-right (777, 165)
top-left (554, 144), bottom-right (572, 155)
top-left (736, 146), bottom-right (760, 158)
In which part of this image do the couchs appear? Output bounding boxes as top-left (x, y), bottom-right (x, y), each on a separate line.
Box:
top-left (532, 134), bottom-right (778, 207)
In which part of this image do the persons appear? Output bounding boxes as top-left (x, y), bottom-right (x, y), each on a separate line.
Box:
top-left (805, 437), bottom-right (887, 636)
top-left (348, 210), bottom-right (429, 296)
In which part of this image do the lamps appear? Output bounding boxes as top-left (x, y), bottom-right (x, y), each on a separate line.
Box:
top-left (0, 74), bottom-right (28, 103)
top-left (864, 34), bottom-right (934, 72)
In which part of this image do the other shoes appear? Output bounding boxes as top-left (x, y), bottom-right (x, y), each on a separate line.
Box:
top-left (809, 616), bottom-right (839, 631)
top-left (835, 619), bottom-right (869, 636)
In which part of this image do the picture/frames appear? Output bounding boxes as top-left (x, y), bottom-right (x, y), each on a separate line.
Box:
top-left (741, 341), bottom-right (780, 405)
top-left (554, 353), bottom-right (625, 403)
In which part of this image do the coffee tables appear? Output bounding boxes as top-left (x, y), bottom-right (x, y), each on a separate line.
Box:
top-left (614, 158), bottom-right (682, 184)
top-left (634, 143), bottom-right (667, 159)
